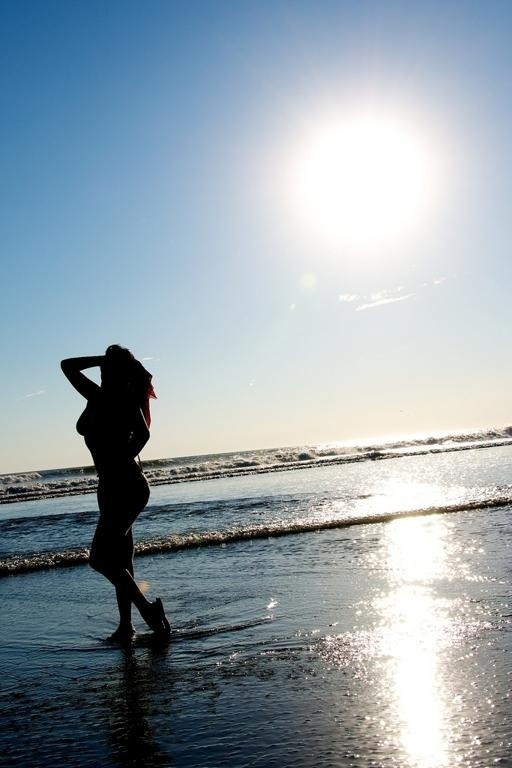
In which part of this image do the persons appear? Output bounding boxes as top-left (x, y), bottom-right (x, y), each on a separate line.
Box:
top-left (61, 344), bottom-right (171, 642)
